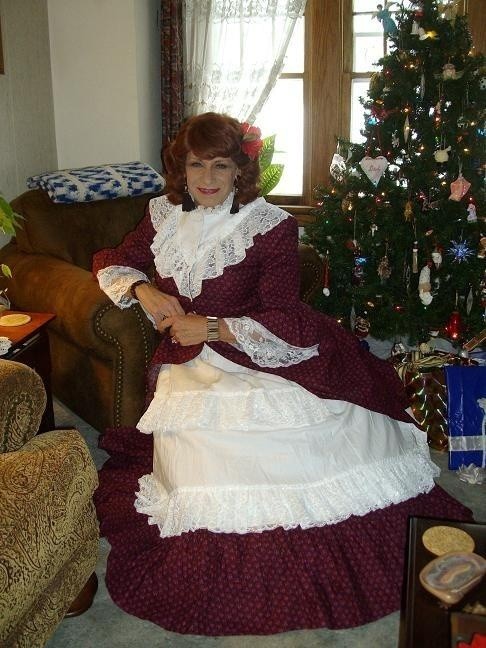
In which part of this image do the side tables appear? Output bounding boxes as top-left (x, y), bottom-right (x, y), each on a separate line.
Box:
top-left (3, 311), bottom-right (75, 449)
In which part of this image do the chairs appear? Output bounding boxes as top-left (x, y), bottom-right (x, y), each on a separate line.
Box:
top-left (2, 170), bottom-right (327, 449)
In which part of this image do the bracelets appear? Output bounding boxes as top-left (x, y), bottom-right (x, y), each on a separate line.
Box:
top-left (130, 280), bottom-right (148, 300)
top-left (206, 315), bottom-right (218, 344)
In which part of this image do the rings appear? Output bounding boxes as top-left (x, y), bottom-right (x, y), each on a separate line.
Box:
top-left (169, 336), bottom-right (176, 341)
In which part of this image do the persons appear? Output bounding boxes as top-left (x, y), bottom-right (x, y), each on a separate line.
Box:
top-left (83, 113), bottom-right (473, 635)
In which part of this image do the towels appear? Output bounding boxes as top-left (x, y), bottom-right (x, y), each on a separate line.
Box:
top-left (26, 161), bottom-right (169, 203)
